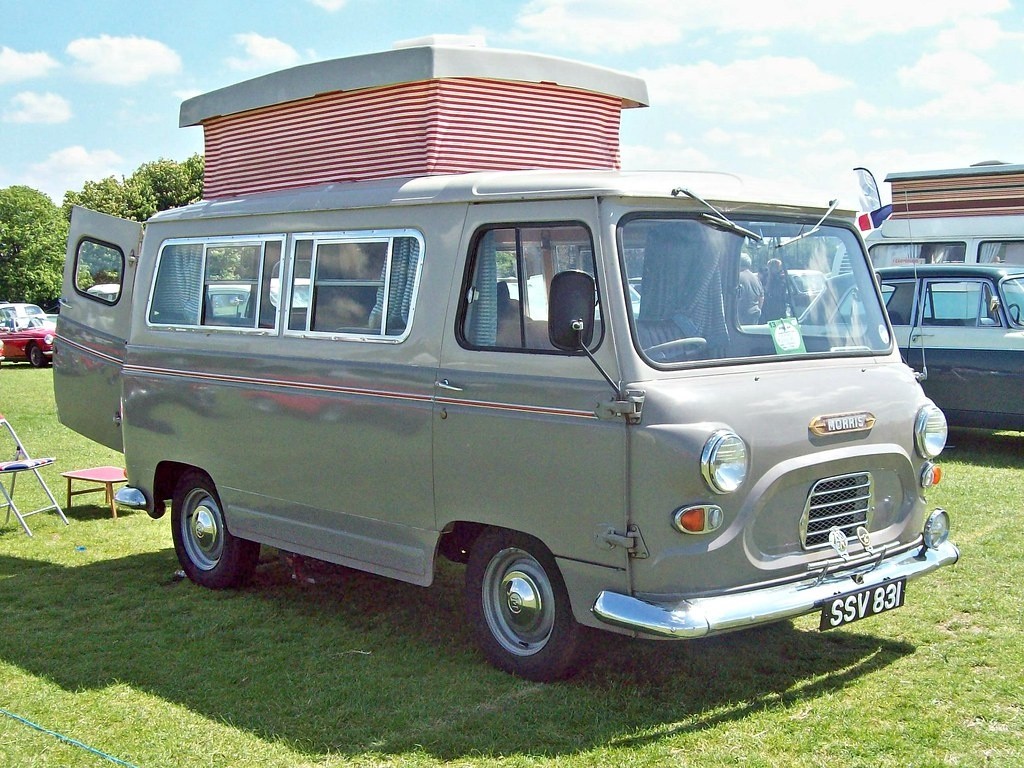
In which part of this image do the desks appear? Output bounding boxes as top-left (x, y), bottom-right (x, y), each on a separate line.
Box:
top-left (61, 467), bottom-right (129, 520)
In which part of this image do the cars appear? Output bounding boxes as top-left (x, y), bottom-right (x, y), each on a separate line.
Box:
top-left (752, 271), bottom-right (828, 319)
top-left (0, 303), bottom-right (48, 325)
top-left (739, 264), bottom-right (1023, 433)
top-left (0, 315), bottom-right (56, 368)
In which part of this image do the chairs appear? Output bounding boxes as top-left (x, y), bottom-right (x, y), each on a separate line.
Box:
top-left (0, 418), bottom-right (70, 536)
top-left (979, 317), bottom-right (996, 326)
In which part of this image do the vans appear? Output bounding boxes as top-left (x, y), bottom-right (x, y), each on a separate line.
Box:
top-left (833, 164), bottom-right (1024, 280)
top-left (51, 45), bottom-right (960, 683)
top-left (204, 278), bottom-right (311, 317)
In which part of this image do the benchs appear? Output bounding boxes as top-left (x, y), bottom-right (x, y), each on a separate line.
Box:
top-left (635, 318), bottom-right (727, 364)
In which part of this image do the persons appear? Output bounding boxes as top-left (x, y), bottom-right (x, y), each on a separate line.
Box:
top-left (737, 253), bottom-right (787, 325)
